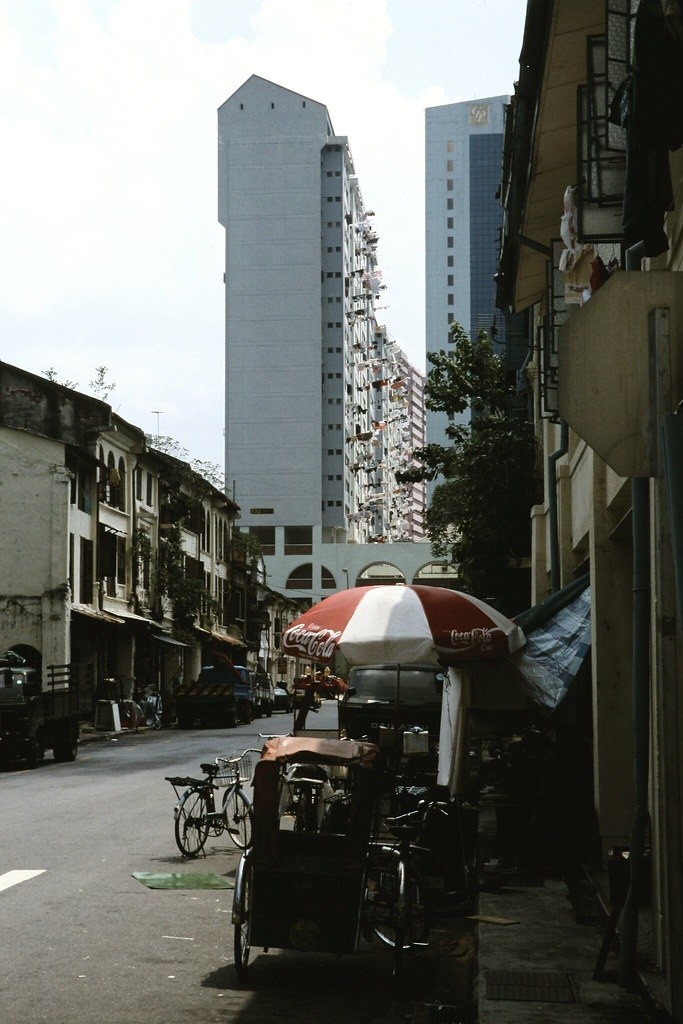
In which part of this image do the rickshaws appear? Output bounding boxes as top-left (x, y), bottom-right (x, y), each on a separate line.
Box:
top-left (226, 733), bottom-right (387, 986)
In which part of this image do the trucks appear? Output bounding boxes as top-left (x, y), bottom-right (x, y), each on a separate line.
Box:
top-left (0, 661), bottom-right (84, 768)
top-left (249, 672), bottom-right (275, 719)
top-left (175, 665), bottom-right (255, 729)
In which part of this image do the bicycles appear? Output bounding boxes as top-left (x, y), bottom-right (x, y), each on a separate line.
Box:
top-left (169, 746), bottom-right (266, 860)
top-left (362, 797), bottom-right (449, 999)
top-left (283, 776), bottom-right (330, 833)
top-left (256, 731), bottom-right (294, 744)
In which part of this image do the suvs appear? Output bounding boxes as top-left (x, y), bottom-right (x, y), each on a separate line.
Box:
top-left (333, 661), bottom-right (449, 777)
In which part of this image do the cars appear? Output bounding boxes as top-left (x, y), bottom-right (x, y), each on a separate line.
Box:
top-left (274, 687), bottom-right (293, 714)
top-left (291, 688), bottom-right (322, 709)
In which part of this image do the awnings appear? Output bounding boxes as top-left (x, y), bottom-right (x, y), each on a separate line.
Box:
top-left (436, 570), bottom-right (592, 717)
top-left (69, 606), bottom-right (247, 649)
top-left (281, 582), bottom-right (528, 781)
